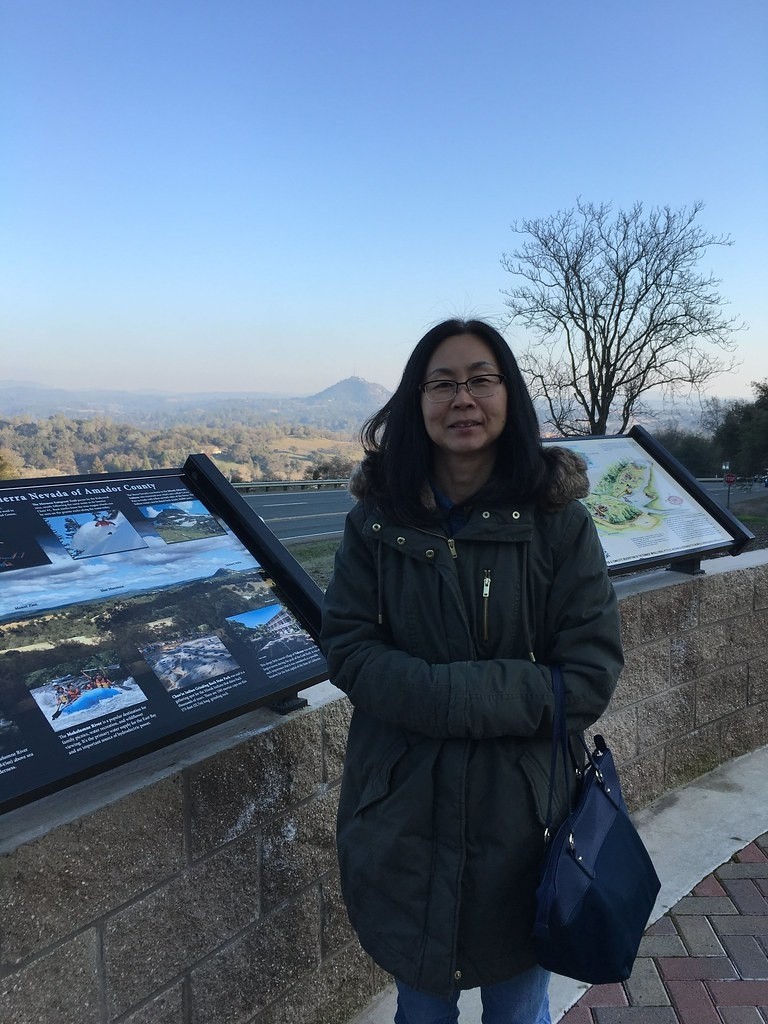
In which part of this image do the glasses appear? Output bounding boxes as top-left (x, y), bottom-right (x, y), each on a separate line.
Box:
top-left (418, 374), bottom-right (506, 403)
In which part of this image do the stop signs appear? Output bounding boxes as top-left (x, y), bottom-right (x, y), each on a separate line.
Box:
top-left (725, 474), bottom-right (737, 484)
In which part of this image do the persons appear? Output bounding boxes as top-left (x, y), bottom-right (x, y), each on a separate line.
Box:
top-left (55, 670), bottom-right (116, 708)
top-left (316, 316), bottom-right (626, 1024)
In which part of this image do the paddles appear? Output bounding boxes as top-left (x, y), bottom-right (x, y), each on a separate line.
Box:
top-left (52, 697), bottom-right (77, 719)
top-left (52, 700), bottom-right (62, 721)
top-left (82, 663), bottom-right (122, 671)
top-left (116, 685), bottom-right (132, 690)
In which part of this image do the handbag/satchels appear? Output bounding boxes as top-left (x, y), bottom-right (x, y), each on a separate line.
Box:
top-left (511, 666), bottom-right (662, 986)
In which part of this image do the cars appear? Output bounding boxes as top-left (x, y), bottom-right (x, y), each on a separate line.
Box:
top-left (764, 476), bottom-right (768, 487)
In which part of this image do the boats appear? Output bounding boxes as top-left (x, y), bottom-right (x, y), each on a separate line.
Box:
top-left (51, 674), bottom-right (74, 685)
top-left (62, 687), bottom-right (122, 714)
